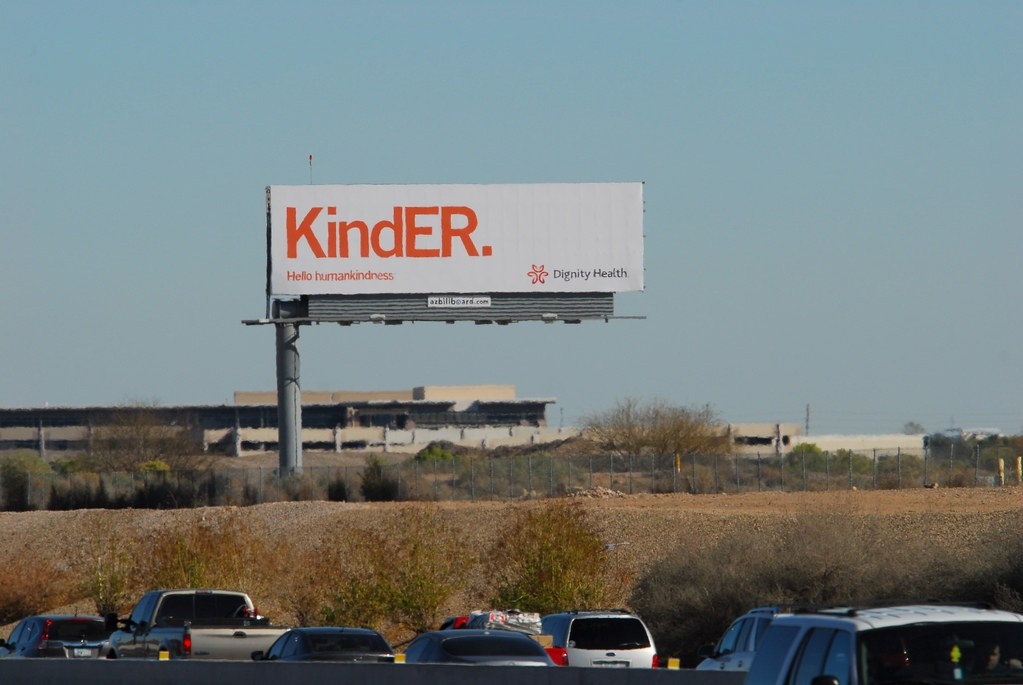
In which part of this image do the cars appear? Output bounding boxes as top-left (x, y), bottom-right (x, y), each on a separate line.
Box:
top-left (399, 628), bottom-right (559, 667)
top-left (0, 611), bottom-right (111, 659)
top-left (251, 625), bottom-right (396, 665)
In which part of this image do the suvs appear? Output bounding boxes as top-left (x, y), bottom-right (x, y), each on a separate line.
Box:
top-left (737, 601), bottom-right (1023, 685)
top-left (539, 607), bottom-right (660, 669)
top-left (690, 604), bottom-right (796, 672)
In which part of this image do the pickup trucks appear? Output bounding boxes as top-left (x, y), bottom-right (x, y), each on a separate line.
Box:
top-left (438, 613), bottom-right (543, 634)
top-left (98, 590), bottom-right (291, 662)
